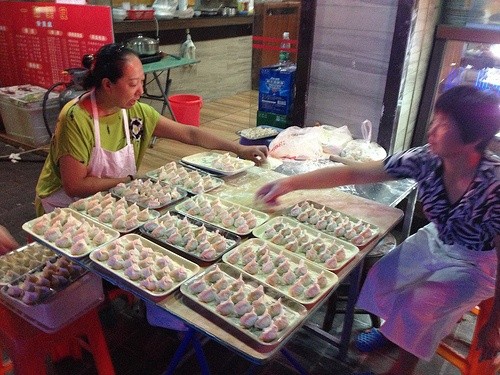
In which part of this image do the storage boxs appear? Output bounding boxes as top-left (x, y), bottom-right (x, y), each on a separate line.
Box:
top-left (0, 101), bottom-right (61, 147)
top-left (256, 63), bottom-right (297, 129)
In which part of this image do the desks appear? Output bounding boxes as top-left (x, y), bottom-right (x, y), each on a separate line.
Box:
top-left (142, 54), bottom-right (200, 123)
top-left (32, 157), bottom-right (426, 375)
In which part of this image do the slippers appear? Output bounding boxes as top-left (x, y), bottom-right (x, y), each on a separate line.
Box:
top-left (354, 327), bottom-right (395, 351)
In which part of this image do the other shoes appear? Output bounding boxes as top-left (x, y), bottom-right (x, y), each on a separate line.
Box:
top-left (112, 292), bottom-right (146, 324)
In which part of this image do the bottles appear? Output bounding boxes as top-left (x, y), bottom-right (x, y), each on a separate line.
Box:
top-left (279, 33), bottom-right (292, 71)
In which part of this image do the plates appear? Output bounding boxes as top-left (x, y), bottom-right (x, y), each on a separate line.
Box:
top-left (0, 239), bottom-right (64, 288)
top-left (182, 151), bottom-right (256, 177)
top-left (69, 192), bottom-right (161, 234)
top-left (179, 261), bottom-right (308, 347)
top-left (0, 84), bottom-right (62, 108)
top-left (109, 177), bottom-right (187, 209)
top-left (0, 254), bottom-right (87, 309)
top-left (236, 123), bottom-right (287, 141)
top-left (173, 192), bottom-right (272, 236)
top-left (221, 237), bottom-right (339, 305)
top-left (281, 201), bottom-right (379, 248)
top-left (179, 147), bottom-right (238, 176)
top-left (89, 230), bottom-right (199, 298)
top-left (143, 162), bottom-right (225, 199)
top-left (138, 210), bottom-right (236, 262)
top-left (252, 214), bottom-right (361, 271)
top-left (21, 207), bottom-right (120, 258)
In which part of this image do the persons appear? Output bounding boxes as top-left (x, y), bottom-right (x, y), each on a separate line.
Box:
top-left (253, 85), bottom-right (500, 375)
top-left (35, 43), bottom-right (268, 323)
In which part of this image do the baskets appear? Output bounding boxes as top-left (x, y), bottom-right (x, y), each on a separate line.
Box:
top-left (126, 9), bottom-right (155, 20)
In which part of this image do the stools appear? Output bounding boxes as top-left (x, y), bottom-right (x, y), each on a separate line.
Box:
top-left (322, 231), bottom-right (396, 333)
top-left (0, 300), bottom-right (115, 375)
top-left (436, 297), bottom-right (500, 375)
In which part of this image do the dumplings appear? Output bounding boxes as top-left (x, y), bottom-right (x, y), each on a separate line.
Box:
top-left (290, 201), bottom-right (372, 244)
top-left (93, 238), bottom-right (189, 292)
top-left (156, 161), bottom-right (257, 233)
top-left (227, 218), bottom-right (345, 299)
top-left (188, 265), bottom-right (289, 342)
top-left (73, 190), bottom-right (151, 230)
top-left (113, 178), bottom-right (180, 208)
top-left (141, 211), bottom-right (227, 259)
top-left (30, 208), bottom-right (106, 256)
top-left (0, 243), bottom-right (83, 306)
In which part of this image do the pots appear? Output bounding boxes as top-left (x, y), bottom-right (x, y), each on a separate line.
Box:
top-left (124, 34), bottom-right (161, 57)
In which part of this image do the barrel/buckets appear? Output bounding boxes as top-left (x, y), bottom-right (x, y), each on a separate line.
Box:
top-left (169, 94), bottom-right (201, 128)
